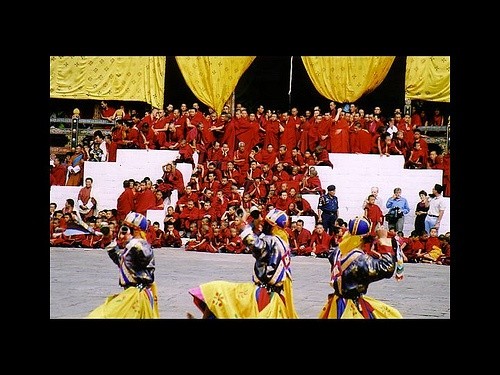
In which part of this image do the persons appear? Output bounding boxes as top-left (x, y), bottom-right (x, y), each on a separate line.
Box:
top-left (188, 207), bottom-right (296, 319)
top-left (316, 214), bottom-right (405, 318)
top-left (51, 98), bottom-right (450, 265)
top-left (84, 211), bottom-right (161, 319)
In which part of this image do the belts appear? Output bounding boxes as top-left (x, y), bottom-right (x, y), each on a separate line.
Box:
top-left (427, 215), bottom-right (439, 217)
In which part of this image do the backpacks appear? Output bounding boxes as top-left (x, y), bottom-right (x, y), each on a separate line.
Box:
top-left (385, 206), bottom-right (403, 223)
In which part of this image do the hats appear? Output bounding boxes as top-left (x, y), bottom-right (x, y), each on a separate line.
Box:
top-left (327, 185), bottom-right (335, 191)
top-left (122, 211), bottom-right (147, 231)
top-left (264, 208), bottom-right (288, 229)
top-left (348, 216), bottom-right (371, 236)
